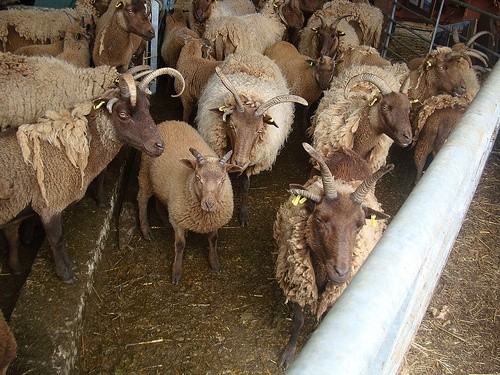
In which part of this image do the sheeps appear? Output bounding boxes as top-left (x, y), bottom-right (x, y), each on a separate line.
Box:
top-left (0, 67), bottom-right (186, 286)
top-left (272, 142), bottom-right (395, 370)
top-left (161, 1), bottom-right (492, 228)
top-left (138, 119), bottom-right (235, 284)
top-left (0, 0), bottom-right (156, 207)
